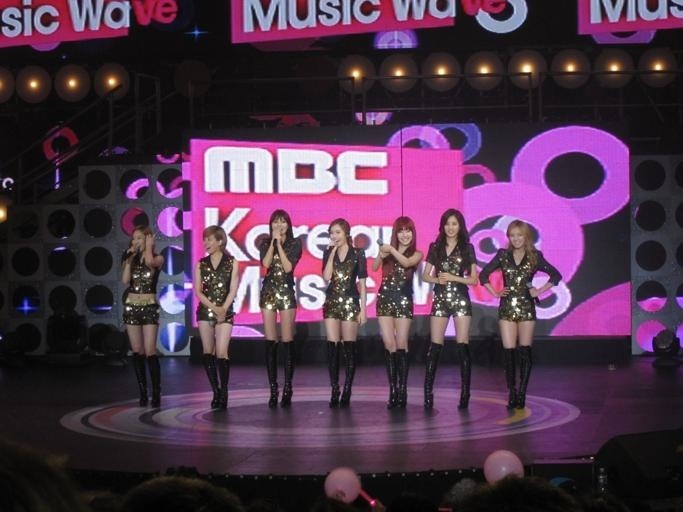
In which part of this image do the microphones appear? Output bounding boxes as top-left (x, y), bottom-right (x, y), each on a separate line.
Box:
top-left (526, 281), bottom-right (541, 308)
top-left (327, 245), bottom-right (334, 255)
top-left (377, 239), bottom-right (384, 247)
top-left (121, 244), bottom-right (140, 263)
top-left (272, 238), bottom-right (284, 244)
top-left (208, 312), bottom-right (237, 328)
top-left (440, 263), bottom-right (450, 292)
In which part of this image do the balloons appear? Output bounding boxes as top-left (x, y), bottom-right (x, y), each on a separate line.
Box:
top-left (324, 467), bottom-right (361, 504)
top-left (483, 449), bottom-right (525, 485)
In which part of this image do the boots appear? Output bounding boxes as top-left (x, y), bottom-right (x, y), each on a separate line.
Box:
top-left (280, 340), bottom-right (295, 407)
top-left (215, 358), bottom-right (231, 410)
top-left (264, 339), bottom-right (280, 407)
top-left (423, 341), bottom-right (445, 409)
top-left (454, 342), bottom-right (472, 409)
top-left (514, 344), bottom-right (534, 409)
top-left (394, 348), bottom-right (410, 408)
top-left (339, 340), bottom-right (358, 408)
top-left (202, 352), bottom-right (221, 408)
top-left (132, 352), bottom-right (149, 408)
top-left (146, 354), bottom-right (162, 408)
top-left (384, 349), bottom-right (399, 409)
top-left (325, 340), bottom-right (342, 408)
top-left (503, 348), bottom-right (518, 408)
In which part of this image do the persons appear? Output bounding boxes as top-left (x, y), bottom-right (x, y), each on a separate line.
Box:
top-left (258, 209), bottom-right (302, 407)
top-left (121, 225), bottom-right (164, 406)
top-left (195, 225), bottom-right (240, 409)
top-left (371, 216), bottom-right (423, 408)
top-left (478, 220), bottom-right (562, 408)
top-left (420, 208), bottom-right (478, 412)
top-left (321, 218), bottom-right (369, 407)
